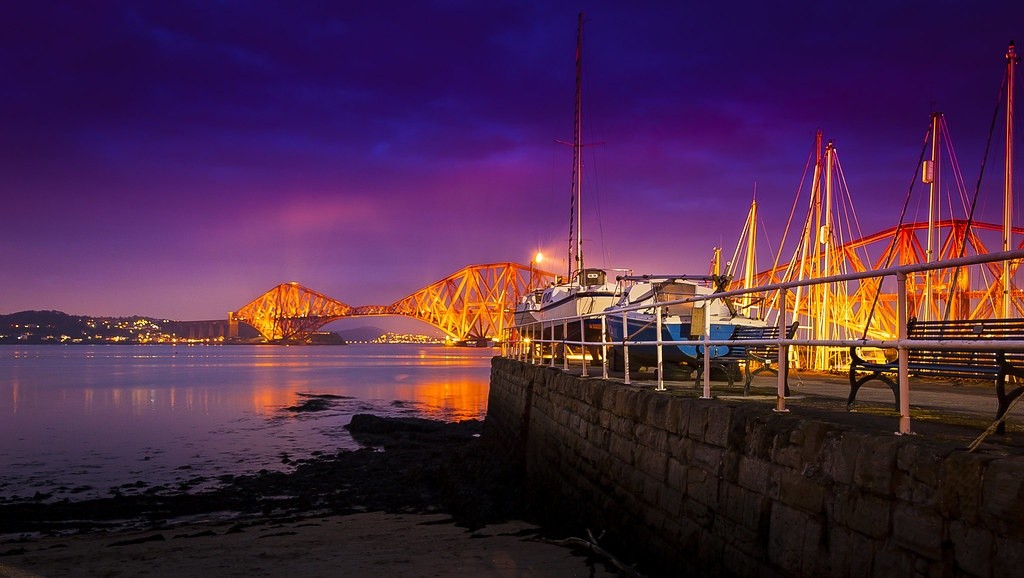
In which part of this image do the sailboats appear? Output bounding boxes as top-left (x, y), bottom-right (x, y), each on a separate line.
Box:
top-left (512, 10), bottom-right (1024, 383)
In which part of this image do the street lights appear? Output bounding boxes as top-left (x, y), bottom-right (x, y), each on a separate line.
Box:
top-left (529, 251), bottom-right (544, 292)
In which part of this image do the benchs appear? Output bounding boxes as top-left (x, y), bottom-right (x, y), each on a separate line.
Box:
top-left (847, 316), bottom-right (1024, 437)
top-left (696, 321), bottom-right (799, 397)
